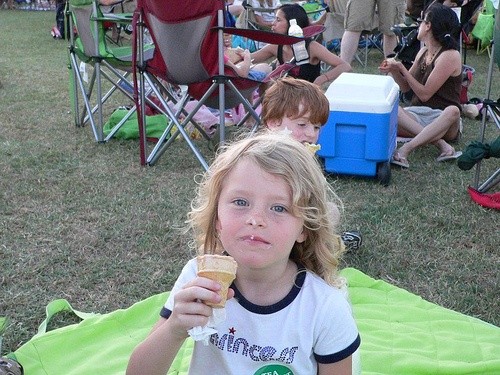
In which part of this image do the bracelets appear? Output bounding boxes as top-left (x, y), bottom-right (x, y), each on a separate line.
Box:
top-left (324, 73), bottom-right (329, 80)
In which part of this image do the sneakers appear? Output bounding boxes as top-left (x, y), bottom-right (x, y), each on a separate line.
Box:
top-left (336, 231), bottom-right (362, 255)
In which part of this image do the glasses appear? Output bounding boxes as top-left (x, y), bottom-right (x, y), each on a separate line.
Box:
top-left (416, 17), bottom-right (429, 25)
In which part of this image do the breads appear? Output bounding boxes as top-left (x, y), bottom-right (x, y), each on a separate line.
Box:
top-left (223, 47), bottom-right (245, 64)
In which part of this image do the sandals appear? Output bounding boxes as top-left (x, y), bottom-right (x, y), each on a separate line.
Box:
top-left (437, 145), bottom-right (463, 161)
top-left (391, 152), bottom-right (410, 168)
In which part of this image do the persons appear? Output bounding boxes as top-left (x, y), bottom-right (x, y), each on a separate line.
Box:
top-left (56, 0), bottom-right (490, 167)
top-left (126, 132), bottom-right (360, 375)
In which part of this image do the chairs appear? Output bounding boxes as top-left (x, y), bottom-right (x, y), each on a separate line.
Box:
top-left (63, 0), bottom-right (500, 194)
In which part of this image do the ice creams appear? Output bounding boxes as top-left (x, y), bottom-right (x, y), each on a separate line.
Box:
top-left (303, 141), bottom-right (321, 155)
top-left (197, 254), bottom-right (238, 308)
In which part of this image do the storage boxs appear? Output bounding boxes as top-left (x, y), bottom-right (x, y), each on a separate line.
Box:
top-left (316, 72), bottom-right (400, 186)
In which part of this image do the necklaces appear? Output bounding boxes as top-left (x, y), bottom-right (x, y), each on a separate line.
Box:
top-left (422, 46), bottom-right (438, 75)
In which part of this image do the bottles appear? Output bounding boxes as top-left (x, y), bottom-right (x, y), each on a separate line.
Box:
top-left (287, 19), bottom-right (310, 65)
